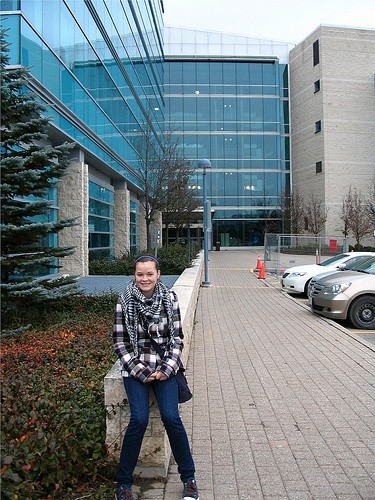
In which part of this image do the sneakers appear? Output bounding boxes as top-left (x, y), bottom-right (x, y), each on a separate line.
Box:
top-left (183, 479), bottom-right (199, 500)
top-left (116, 484), bottom-right (132, 500)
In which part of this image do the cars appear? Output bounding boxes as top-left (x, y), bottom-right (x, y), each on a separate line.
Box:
top-left (280, 252), bottom-right (375, 299)
top-left (307, 255), bottom-right (373, 300)
top-left (311, 256), bottom-right (375, 331)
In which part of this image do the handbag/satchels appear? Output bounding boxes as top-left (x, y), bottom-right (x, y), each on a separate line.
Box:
top-left (175, 366), bottom-right (193, 403)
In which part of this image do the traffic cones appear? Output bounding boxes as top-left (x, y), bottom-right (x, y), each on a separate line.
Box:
top-left (255, 258), bottom-right (263, 269)
top-left (256, 262), bottom-right (265, 279)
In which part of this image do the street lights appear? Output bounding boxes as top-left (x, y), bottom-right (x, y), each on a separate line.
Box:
top-left (197, 158), bottom-right (214, 285)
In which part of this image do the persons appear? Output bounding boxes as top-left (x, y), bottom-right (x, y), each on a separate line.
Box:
top-left (112, 256), bottom-right (198, 500)
